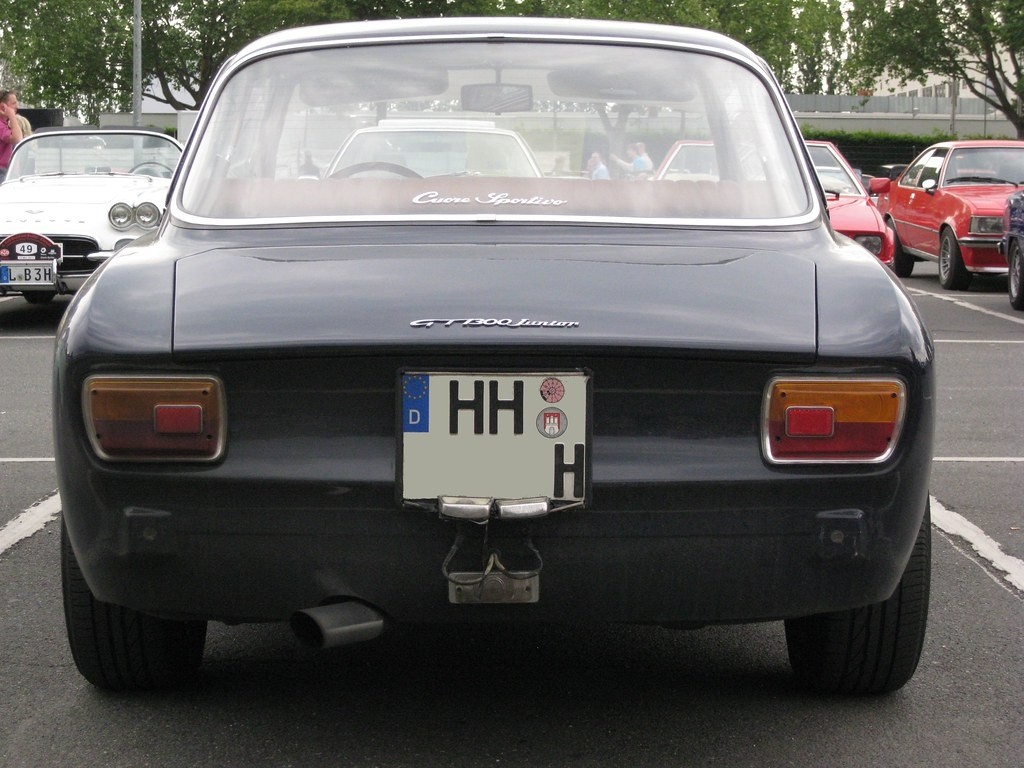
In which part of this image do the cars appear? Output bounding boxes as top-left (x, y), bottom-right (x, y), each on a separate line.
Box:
top-left (646, 136), bottom-right (901, 278)
top-left (997, 189), bottom-right (1023, 312)
top-left (54, 18), bottom-right (940, 703)
top-left (871, 137), bottom-right (1024, 292)
top-left (0, 128), bottom-right (183, 310)
top-left (325, 117), bottom-right (541, 190)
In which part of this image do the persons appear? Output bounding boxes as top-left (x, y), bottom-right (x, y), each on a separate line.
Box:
top-left (611, 142), bottom-right (653, 172)
top-left (588, 151), bottom-right (610, 180)
top-left (0, 90), bottom-right (31, 183)
